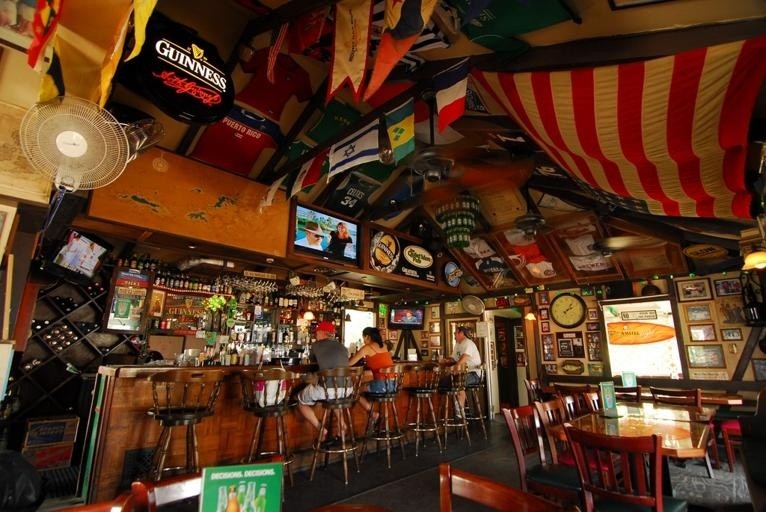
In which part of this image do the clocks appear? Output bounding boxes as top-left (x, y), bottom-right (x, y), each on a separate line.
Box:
top-left (549, 293), bottom-right (587, 329)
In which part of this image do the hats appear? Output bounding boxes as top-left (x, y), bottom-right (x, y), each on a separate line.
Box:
top-left (312, 321), bottom-right (336, 333)
top-left (302, 221), bottom-right (326, 238)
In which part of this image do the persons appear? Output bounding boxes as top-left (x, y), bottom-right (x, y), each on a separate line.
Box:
top-left (441, 325), bottom-right (482, 422)
top-left (79, 242), bottom-right (95, 268)
top-left (293, 221), bottom-right (325, 251)
top-left (325, 221), bottom-right (353, 257)
top-left (55, 234), bottom-right (82, 266)
top-left (348, 326), bottom-right (398, 427)
top-left (297, 321), bottom-right (354, 442)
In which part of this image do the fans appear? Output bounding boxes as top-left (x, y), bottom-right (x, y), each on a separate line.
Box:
top-left (18, 95), bottom-right (129, 195)
top-left (462, 295), bottom-right (485, 316)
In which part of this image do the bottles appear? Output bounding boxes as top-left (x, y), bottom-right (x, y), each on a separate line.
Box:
top-left (1, 385), bottom-right (22, 421)
top-left (30, 319), bottom-right (49, 331)
top-left (155, 268), bottom-right (210, 290)
top-left (279, 286), bottom-right (346, 310)
top-left (311, 313), bottom-right (341, 327)
top-left (77, 322), bottom-right (98, 334)
top-left (100, 346), bottom-right (110, 354)
top-left (211, 310), bottom-right (297, 345)
top-left (215, 275), bottom-right (278, 306)
top-left (435, 348), bottom-right (439, 362)
top-left (23, 357), bottom-right (41, 370)
top-left (86, 283), bottom-right (103, 296)
top-left (297, 328), bottom-right (316, 366)
top-left (199, 345), bottom-right (289, 367)
top-left (117, 253), bottom-right (158, 275)
top-left (215, 481), bottom-right (267, 512)
top-left (43, 324), bottom-right (77, 349)
top-left (355, 339), bottom-right (361, 354)
top-left (172, 309), bottom-right (214, 330)
top-left (55, 295), bottom-right (77, 313)
top-left (153, 318), bottom-right (171, 331)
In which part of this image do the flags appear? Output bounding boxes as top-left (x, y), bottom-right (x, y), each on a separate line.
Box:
top-left (432, 60), bottom-right (469, 136)
top-left (257, 173), bottom-right (288, 215)
top-left (325, 117), bottom-right (380, 179)
top-left (286, 145), bottom-right (330, 200)
top-left (472, 16), bottom-right (766, 222)
top-left (265, 2), bottom-right (292, 84)
top-left (382, 97), bottom-right (416, 166)
top-left (324, 0), bottom-right (372, 106)
top-left (362, 0), bottom-right (438, 99)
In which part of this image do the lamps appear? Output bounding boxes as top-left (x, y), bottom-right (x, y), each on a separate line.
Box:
top-left (742, 243), bottom-right (766, 271)
top-left (525, 309), bottom-right (536, 321)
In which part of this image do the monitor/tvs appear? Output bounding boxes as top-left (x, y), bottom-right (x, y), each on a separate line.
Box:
top-left (294, 205), bottom-right (365, 268)
top-left (49, 228), bottom-right (113, 282)
top-left (388, 307), bottom-right (425, 331)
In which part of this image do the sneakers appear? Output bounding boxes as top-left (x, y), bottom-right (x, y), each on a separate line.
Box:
top-left (447, 415), bottom-right (464, 424)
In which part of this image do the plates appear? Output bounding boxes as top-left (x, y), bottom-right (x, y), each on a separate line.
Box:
top-left (273, 357), bottom-right (302, 364)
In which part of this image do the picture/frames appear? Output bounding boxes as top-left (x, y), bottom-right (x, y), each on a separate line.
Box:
top-left (585, 332), bottom-right (602, 361)
top-left (687, 323), bottom-right (717, 342)
top-left (432, 348), bottom-right (442, 362)
top-left (421, 341), bottom-right (428, 348)
top-left (379, 318), bottom-right (385, 328)
top-left (713, 277), bottom-right (745, 297)
top-left (580, 287), bottom-right (595, 296)
top-left (514, 326), bottom-right (523, 337)
top-left (752, 357), bottom-right (766, 381)
top-left (541, 322), bottom-right (550, 332)
top-left (558, 338), bottom-right (574, 357)
top-left (388, 329), bottom-right (398, 341)
top-left (682, 304), bottom-right (715, 322)
top-left (447, 317), bottom-right (483, 363)
top-left (720, 328), bottom-right (743, 341)
top-left (586, 322), bottom-right (600, 330)
top-left (428, 321), bottom-right (440, 333)
top-left (421, 331), bottom-right (428, 339)
top-left (431, 306), bottom-right (440, 319)
top-left (545, 364), bottom-right (557, 373)
top-left (541, 334), bottom-right (556, 361)
top-left (539, 308), bottom-right (549, 320)
top-left (420, 349), bottom-right (428, 356)
top-left (685, 344), bottom-right (727, 368)
top-left (537, 291), bottom-right (550, 305)
top-left (430, 335), bottom-right (440, 347)
top-left (515, 337), bottom-right (525, 349)
top-left (515, 352), bottom-right (526, 367)
top-left (589, 308), bottom-right (597, 319)
top-left (675, 277), bottom-right (714, 302)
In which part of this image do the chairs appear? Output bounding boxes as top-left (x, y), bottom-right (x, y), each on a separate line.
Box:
top-left (650, 386), bottom-right (721, 479)
top-left (721, 390), bottom-right (766, 470)
top-left (614, 385), bottom-right (641, 402)
top-left (583, 392), bottom-right (604, 411)
top-left (561, 392), bottom-right (588, 419)
top-left (404, 361), bottom-right (446, 457)
top-left (523, 377), bottom-right (545, 405)
top-left (308, 367), bottom-right (363, 484)
top-left (364, 363), bottom-right (412, 468)
top-left (464, 361), bottom-right (488, 440)
top-left (501, 404), bottom-right (601, 508)
top-left (562, 422), bottom-right (691, 512)
top-left (434, 361), bottom-right (471, 450)
top-left (238, 366), bottom-right (298, 502)
top-left (147, 370), bottom-right (224, 482)
top-left (553, 383), bottom-right (592, 405)
top-left (438, 463), bottom-right (582, 512)
top-left (533, 398), bottom-right (626, 490)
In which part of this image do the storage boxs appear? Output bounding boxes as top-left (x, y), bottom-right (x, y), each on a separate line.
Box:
top-left (23, 414), bottom-right (80, 447)
top-left (22, 444), bottom-right (73, 471)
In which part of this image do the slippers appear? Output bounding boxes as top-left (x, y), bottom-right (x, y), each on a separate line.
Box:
top-left (334, 432), bottom-right (351, 442)
top-left (370, 413), bottom-right (385, 431)
top-left (314, 438), bottom-right (333, 448)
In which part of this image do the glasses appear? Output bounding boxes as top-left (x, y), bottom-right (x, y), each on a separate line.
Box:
top-left (315, 233), bottom-right (325, 239)
